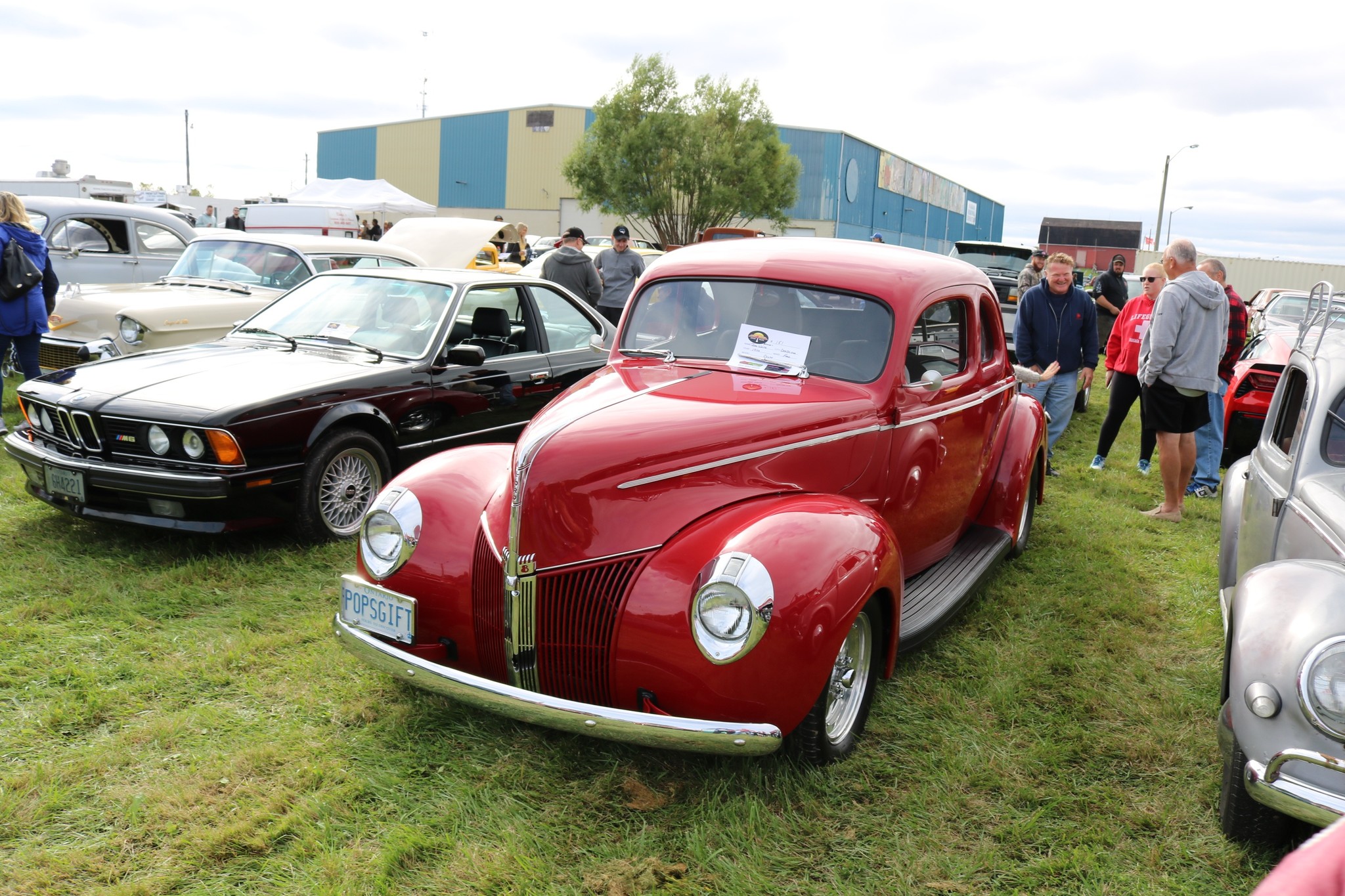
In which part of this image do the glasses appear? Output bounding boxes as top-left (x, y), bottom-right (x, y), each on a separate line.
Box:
top-left (1160, 257), bottom-right (1173, 264)
top-left (1140, 276), bottom-right (1161, 283)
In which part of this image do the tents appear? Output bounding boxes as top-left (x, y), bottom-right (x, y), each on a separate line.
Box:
top-left (286, 177), bottom-right (436, 237)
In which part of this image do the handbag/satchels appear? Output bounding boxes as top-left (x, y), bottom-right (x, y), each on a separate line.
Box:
top-left (0, 225), bottom-right (44, 301)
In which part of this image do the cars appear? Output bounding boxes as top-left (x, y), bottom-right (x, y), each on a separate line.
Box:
top-left (330, 228), bottom-right (1052, 765)
top-left (0, 208), bottom-right (1345, 468)
top-left (1213, 278), bottom-right (1345, 845)
top-left (0, 265), bottom-right (676, 552)
top-left (8, 217), bottom-right (522, 381)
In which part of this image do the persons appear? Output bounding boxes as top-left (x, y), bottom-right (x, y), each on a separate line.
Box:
top-left (195, 205), bottom-right (217, 228)
top-left (1013, 239), bottom-right (1249, 522)
top-left (0, 191), bottom-right (59, 434)
top-left (488, 215), bottom-right (531, 267)
top-left (870, 232), bottom-right (884, 243)
top-left (225, 207), bottom-right (246, 231)
top-left (539, 225), bottom-right (716, 339)
top-left (356, 215), bottom-right (393, 241)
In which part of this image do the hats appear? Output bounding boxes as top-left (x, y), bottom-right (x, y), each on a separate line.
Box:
top-left (612, 225), bottom-right (630, 240)
top-left (1112, 255), bottom-right (1125, 264)
top-left (562, 227), bottom-right (590, 245)
top-left (870, 233), bottom-right (882, 238)
top-left (494, 215), bottom-right (504, 220)
top-left (1032, 249), bottom-right (1048, 258)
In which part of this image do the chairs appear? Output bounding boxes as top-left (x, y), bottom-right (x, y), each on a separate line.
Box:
top-left (459, 307), bottom-right (519, 358)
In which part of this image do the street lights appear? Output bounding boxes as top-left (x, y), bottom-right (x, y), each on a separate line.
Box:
top-left (1152, 143), bottom-right (1200, 251)
top-left (1166, 205), bottom-right (1194, 246)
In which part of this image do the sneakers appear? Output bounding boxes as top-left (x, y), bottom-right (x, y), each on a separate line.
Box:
top-left (1090, 454), bottom-right (1106, 471)
top-left (1137, 459), bottom-right (1152, 475)
top-left (1183, 482), bottom-right (1218, 498)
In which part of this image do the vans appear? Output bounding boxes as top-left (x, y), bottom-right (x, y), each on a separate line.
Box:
top-left (0, 195), bottom-right (201, 292)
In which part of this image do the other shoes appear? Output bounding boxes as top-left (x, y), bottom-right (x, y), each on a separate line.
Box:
top-left (1139, 502), bottom-right (1185, 523)
top-left (1046, 459), bottom-right (1061, 477)
top-left (13, 420), bottom-right (31, 430)
top-left (0, 417), bottom-right (8, 436)
